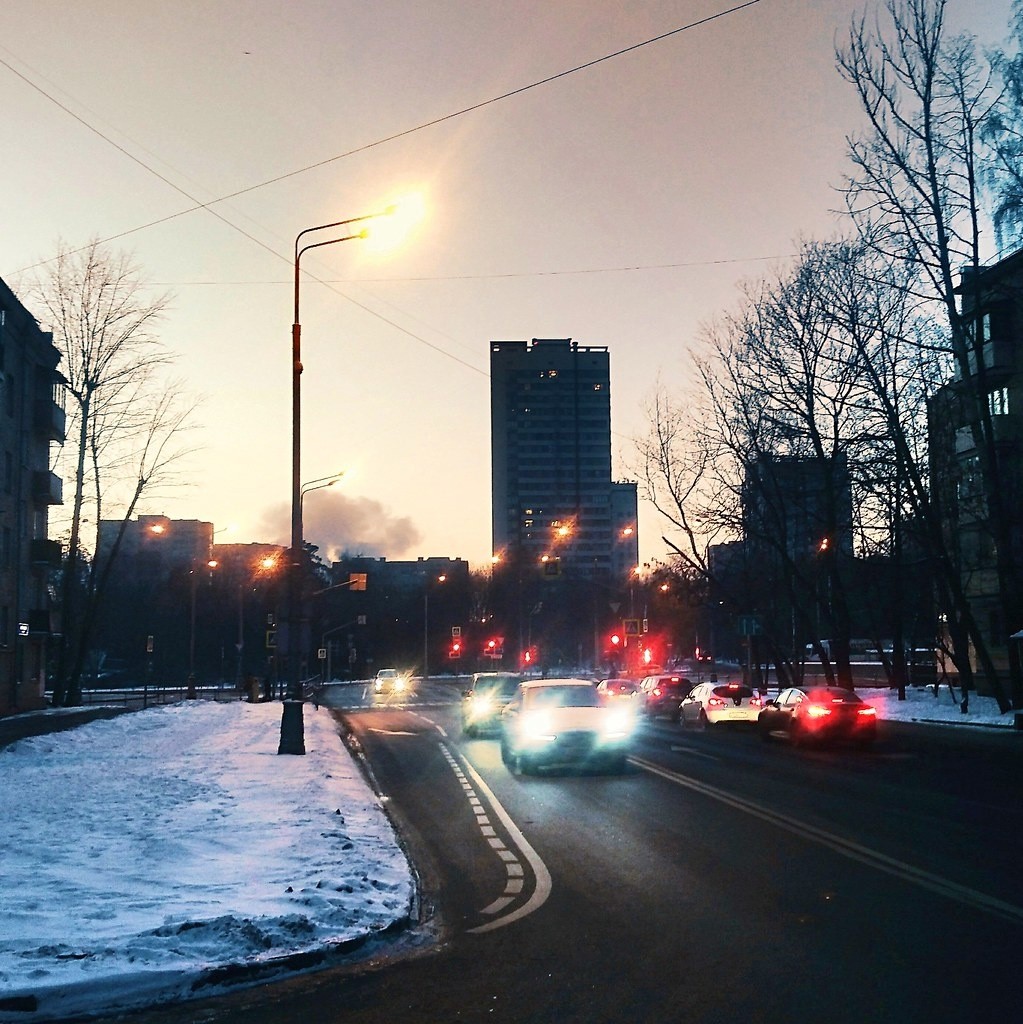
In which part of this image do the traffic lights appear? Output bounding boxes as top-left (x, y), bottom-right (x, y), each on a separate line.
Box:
top-left (645, 648), bottom-right (651, 662)
top-left (488, 640), bottom-right (495, 648)
top-left (453, 641), bottom-right (459, 653)
top-left (610, 633), bottom-right (621, 647)
top-left (526, 652), bottom-right (530, 662)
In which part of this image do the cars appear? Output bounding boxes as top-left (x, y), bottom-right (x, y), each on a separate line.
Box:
top-left (759, 685), bottom-right (878, 748)
top-left (374, 669), bottom-right (404, 694)
top-left (641, 675), bottom-right (693, 723)
top-left (596, 679), bottom-right (638, 704)
top-left (499, 675), bottom-right (627, 778)
top-left (460, 673), bottom-right (523, 738)
top-left (678, 682), bottom-right (761, 734)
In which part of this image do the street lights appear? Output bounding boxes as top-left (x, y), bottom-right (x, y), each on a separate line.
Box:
top-left (301, 469), bottom-right (347, 527)
top-left (276, 191), bottom-right (429, 754)
